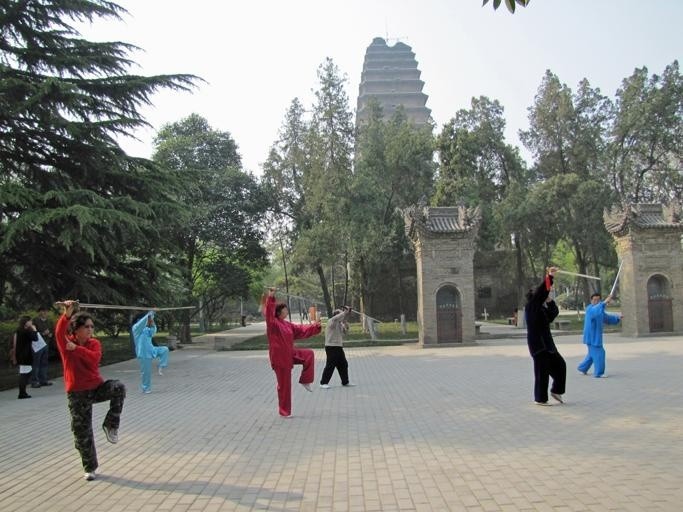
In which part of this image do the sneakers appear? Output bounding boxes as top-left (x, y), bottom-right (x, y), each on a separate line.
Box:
top-left (18, 381), bottom-right (53, 399)
top-left (142, 367), bottom-right (164, 395)
top-left (550, 392), bottom-right (564, 404)
top-left (84, 469), bottom-right (96, 480)
top-left (299, 383), bottom-right (356, 392)
top-left (533, 400), bottom-right (553, 407)
top-left (576, 367), bottom-right (608, 378)
top-left (102, 424), bottom-right (119, 444)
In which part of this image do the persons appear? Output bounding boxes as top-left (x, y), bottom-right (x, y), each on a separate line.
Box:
top-left (131, 308), bottom-right (169, 394)
top-left (31, 305), bottom-right (53, 388)
top-left (56, 300), bottom-right (126, 480)
top-left (264, 287), bottom-right (322, 418)
top-left (575, 292), bottom-right (624, 379)
top-left (318, 306), bottom-right (356, 389)
top-left (12, 315), bottom-right (39, 400)
top-left (302, 304), bottom-right (308, 320)
top-left (524, 265), bottom-right (566, 406)
